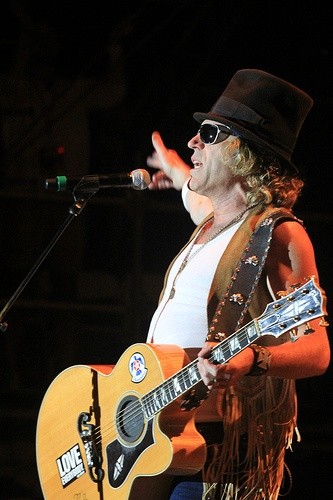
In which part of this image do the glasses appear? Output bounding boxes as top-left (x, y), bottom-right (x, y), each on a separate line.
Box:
top-left (195, 123), bottom-right (237, 145)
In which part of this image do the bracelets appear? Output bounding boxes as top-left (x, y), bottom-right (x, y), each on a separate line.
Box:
top-left (247, 342), bottom-right (272, 379)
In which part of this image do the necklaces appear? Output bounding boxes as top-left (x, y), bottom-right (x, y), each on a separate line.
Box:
top-left (149, 197), bottom-right (257, 345)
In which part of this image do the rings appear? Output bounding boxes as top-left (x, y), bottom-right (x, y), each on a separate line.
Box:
top-left (214, 373), bottom-right (233, 385)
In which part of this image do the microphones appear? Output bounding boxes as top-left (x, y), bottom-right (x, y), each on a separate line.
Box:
top-left (45, 168), bottom-right (150, 191)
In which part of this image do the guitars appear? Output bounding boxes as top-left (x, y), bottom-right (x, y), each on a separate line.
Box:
top-left (36, 275), bottom-right (329, 500)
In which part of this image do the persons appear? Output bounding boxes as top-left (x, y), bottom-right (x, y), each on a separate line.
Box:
top-left (146, 67), bottom-right (330, 500)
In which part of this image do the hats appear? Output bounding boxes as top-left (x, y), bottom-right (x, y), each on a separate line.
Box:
top-left (192, 68), bottom-right (314, 176)
